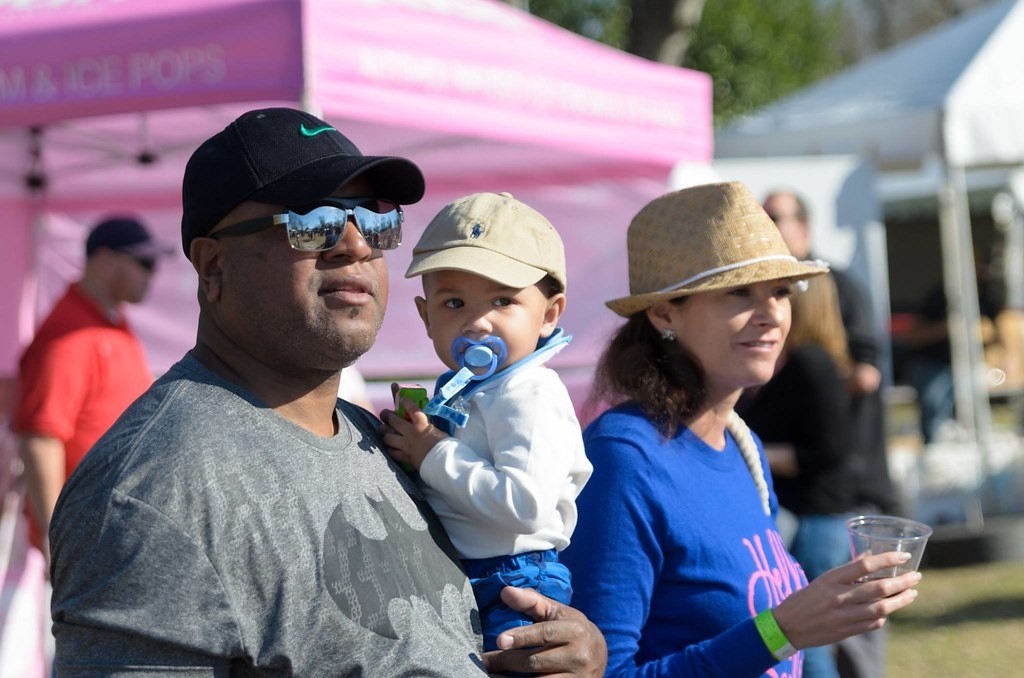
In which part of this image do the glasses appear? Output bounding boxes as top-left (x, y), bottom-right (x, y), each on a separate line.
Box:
top-left (206, 195), bottom-right (404, 255)
top-left (106, 244), bottom-right (158, 273)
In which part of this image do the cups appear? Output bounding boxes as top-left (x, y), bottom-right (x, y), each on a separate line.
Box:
top-left (845, 514), bottom-right (934, 586)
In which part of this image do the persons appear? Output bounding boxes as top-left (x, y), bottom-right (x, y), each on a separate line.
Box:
top-left (741, 255), bottom-right (868, 674)
top-left (47, 110), bottom-right (609, 678)
top-left (380, 184), bottom-right (597, 653)
top-left (568, 180), bottom-right (921, 678)
top-left (14, 214), bottom-right (176, 551)
top-left (747, 187), bottom-right (905, 525)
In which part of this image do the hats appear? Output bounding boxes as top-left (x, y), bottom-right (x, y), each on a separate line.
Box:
top-left (602, 179), bottom-right (830, 316)
top-left (77, 213), bottom-right (181, 262)
top-left (405, 185), bottom-right (568, 299)
top-left (178, 105), bottom-right (427, 261)
